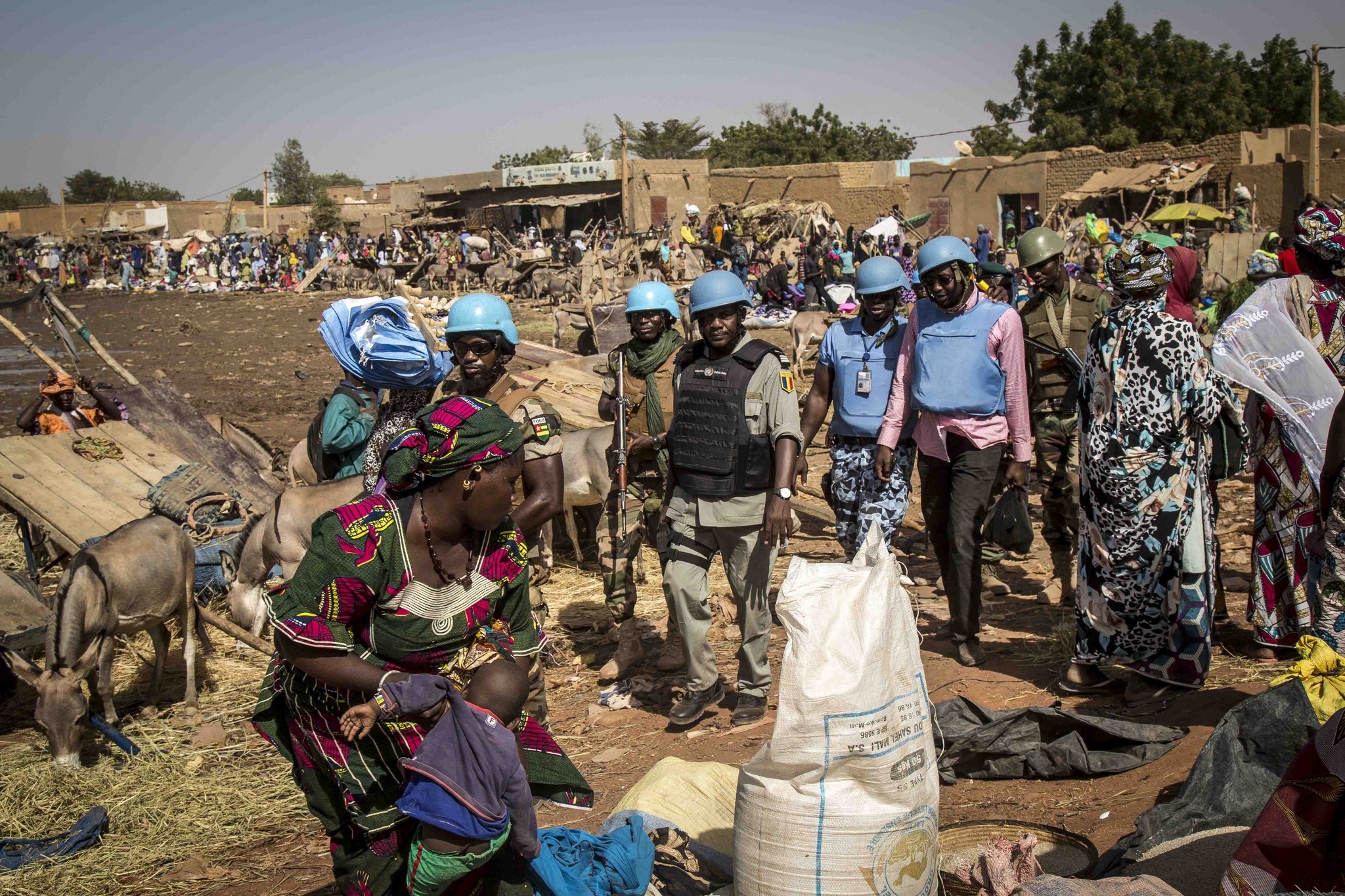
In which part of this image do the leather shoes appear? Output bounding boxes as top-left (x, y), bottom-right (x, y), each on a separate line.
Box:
top-left (957, 640), bottom-right (984, 666)
top-left (935, 619), bottom-right (953, 640)
top-left (731, 693), bottom-right (768, 724)
top-left (666, 679), bottom-right (725, 724)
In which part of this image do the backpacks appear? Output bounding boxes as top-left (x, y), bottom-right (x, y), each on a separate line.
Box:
top-left (306, 387), bottom-right (371, 482)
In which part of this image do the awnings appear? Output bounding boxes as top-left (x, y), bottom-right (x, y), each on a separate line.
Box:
top-left (482, 191), bottom-right (621, 208)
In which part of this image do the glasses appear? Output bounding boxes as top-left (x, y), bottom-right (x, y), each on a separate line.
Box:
top-left (924, 275), bottom-right (955, 287)
top-left (451, 341), bottom-right (494, 355)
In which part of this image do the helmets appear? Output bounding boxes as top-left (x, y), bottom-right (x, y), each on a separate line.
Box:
top-left (625, 281), bottom-right (681, 324)
top-left (916, 235), bottom-right (978, 289)
top-left (688, 270), bottom-right (754, 323)
top-left (854, 256), bottom-right (911, 299)
top-left (1017, 227), bottom-right (1066, 269)
top-left (444, 293), bottom-right (518, 349)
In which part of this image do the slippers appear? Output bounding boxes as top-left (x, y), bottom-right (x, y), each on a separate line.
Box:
top-left (1127, 684), bottom-right (1183, 708)
top-left (1244, 644), bottom-right (1279, 663)
top-left (1057, 678), bottom-right (1121, 694)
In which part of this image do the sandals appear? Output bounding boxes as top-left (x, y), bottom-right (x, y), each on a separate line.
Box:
top-left (978, 561), bottom-right (1011, 594)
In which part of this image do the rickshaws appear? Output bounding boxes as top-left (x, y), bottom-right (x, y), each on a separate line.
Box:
top-left (507, 214), bottom-right (678, 298)
top-left (0, 269), bottom-right (281, 659)
top-left (340, 238), bottom-right (383, 270)
top-left (405, 229), bottom-right (462, 291)
top-left (0, 568), bottom-right (143, 755)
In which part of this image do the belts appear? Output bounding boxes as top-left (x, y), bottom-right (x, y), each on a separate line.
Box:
top-left (842, 435), bottom-right (908, 444)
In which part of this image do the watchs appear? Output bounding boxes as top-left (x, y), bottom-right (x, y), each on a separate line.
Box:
top-left (772, 487), bottom-right (792, 500)
top-left (651, 435), bottom-right (662, 450)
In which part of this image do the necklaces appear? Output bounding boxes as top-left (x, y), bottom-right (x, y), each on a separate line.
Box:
top-left (419, 489), bottom-right (475, 587)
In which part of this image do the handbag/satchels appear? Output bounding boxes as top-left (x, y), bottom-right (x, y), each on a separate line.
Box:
top-left (148, 463), bottom-right (252, 526)
top-left (529, 587), bottom-right (551, 628)
top-left (982, 481), bottom-right (1034, 553)
top-left (1210, 409), bottom-right (1241, 483)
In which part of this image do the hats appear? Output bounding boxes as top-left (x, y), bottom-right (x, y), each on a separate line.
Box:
top-left (978, 224), bottom-right (985, 232)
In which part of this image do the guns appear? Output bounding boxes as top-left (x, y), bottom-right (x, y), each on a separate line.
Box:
top-left (617, 350), bottom-right (627, 542)
top-left (1054, 346), bottom-right (1085, 384)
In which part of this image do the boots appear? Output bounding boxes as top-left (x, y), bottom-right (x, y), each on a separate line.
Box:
top-left (656, 617), bottom-right (687, 670)
top-left (936, 577), bottom-right (945, 591)
top-left (599, 615), bottom-right (645, 679)
top-left (1035, 551), bottom-right (1074, 603)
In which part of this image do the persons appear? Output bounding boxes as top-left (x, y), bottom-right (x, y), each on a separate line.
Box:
top-left (599, 280), bottom-right (687, 685)
top-left (1019, 226), bottom-right (1114, 611)
top-left (598, 204), bottom-right (993, 307)
top-left (16, 230), bottom-right (589, 290)
top-left (657, 270), bottom-right (805, 725)
top-left (995, 200), bottom-right (1345, 304)
top-left (874, 236), bottom-right (1031, 666)
top-left (858, 382), bottom-right (863, 390)
top-left (16, 369), bottom-right (122, 435)
top-left (791, 256), bottom-right (1012, 562)
top-left (1059, 233), bottom-right (1250, 706)
top-left (1211, 207), bottom-right (1345, 663)
top-left (250, 290), bottom-right (595, 896)
top-left (512, 218), bottom-right (523, 233)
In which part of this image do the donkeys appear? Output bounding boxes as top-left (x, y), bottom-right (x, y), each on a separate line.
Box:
top-left (789, 310), bottom-right (831, 382)
top-left (206, 414), bottom-right (614, 639)
top-left (1063, 234), bottom-right (1091, 264)
top-left (326, 261), bottom-right (666, 349)
top-left (0, 515), bottom-right (214, 770)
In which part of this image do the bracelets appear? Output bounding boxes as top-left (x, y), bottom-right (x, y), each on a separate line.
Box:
top-left (377, 670), bottom-right (401, 692)
